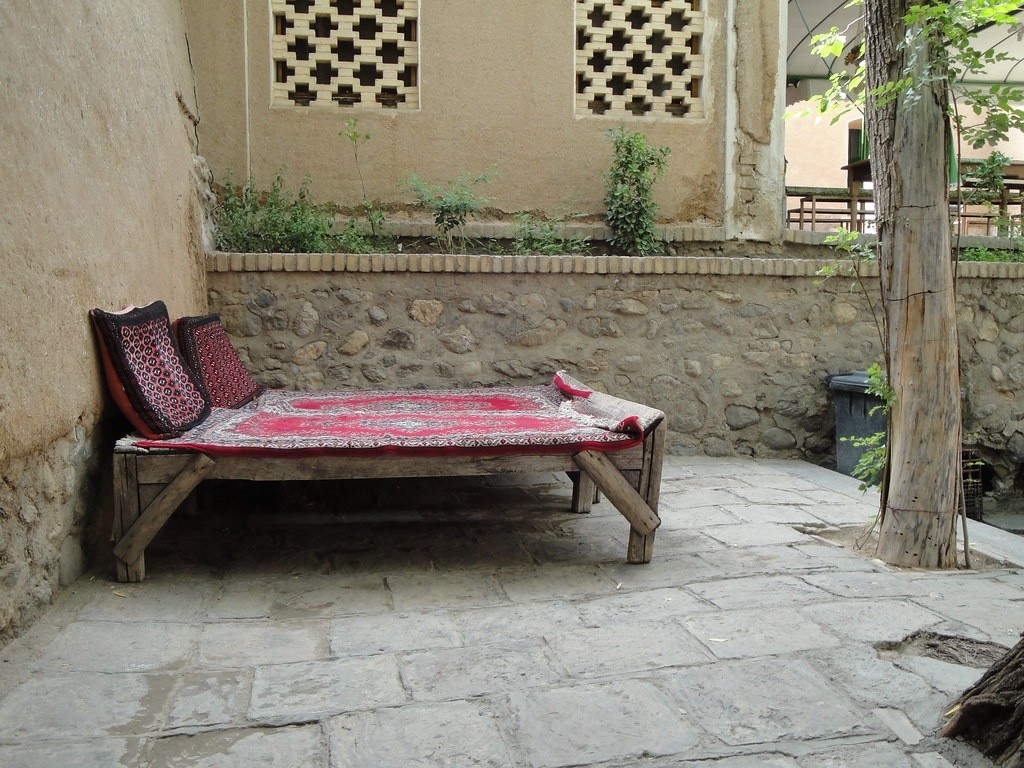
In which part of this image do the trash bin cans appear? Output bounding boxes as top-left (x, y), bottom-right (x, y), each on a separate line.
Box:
top-left (826, 370), bottom-right (888, 487)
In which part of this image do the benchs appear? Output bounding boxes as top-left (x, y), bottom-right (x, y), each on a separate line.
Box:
top-left (785, 176), bottom-right (874, 232)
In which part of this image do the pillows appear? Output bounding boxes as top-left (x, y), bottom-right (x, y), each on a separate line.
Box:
top-left (90, 300), bottom-right (212, 439)
top-left (172, 312), bottom-right (266, 410)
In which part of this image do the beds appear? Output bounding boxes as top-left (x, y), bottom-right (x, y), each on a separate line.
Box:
top-left (110, 366), bottom-right (669, 583)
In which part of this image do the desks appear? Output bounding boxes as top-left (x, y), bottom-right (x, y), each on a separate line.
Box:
top-left (841, 156), bottom-right (1024, 235)
